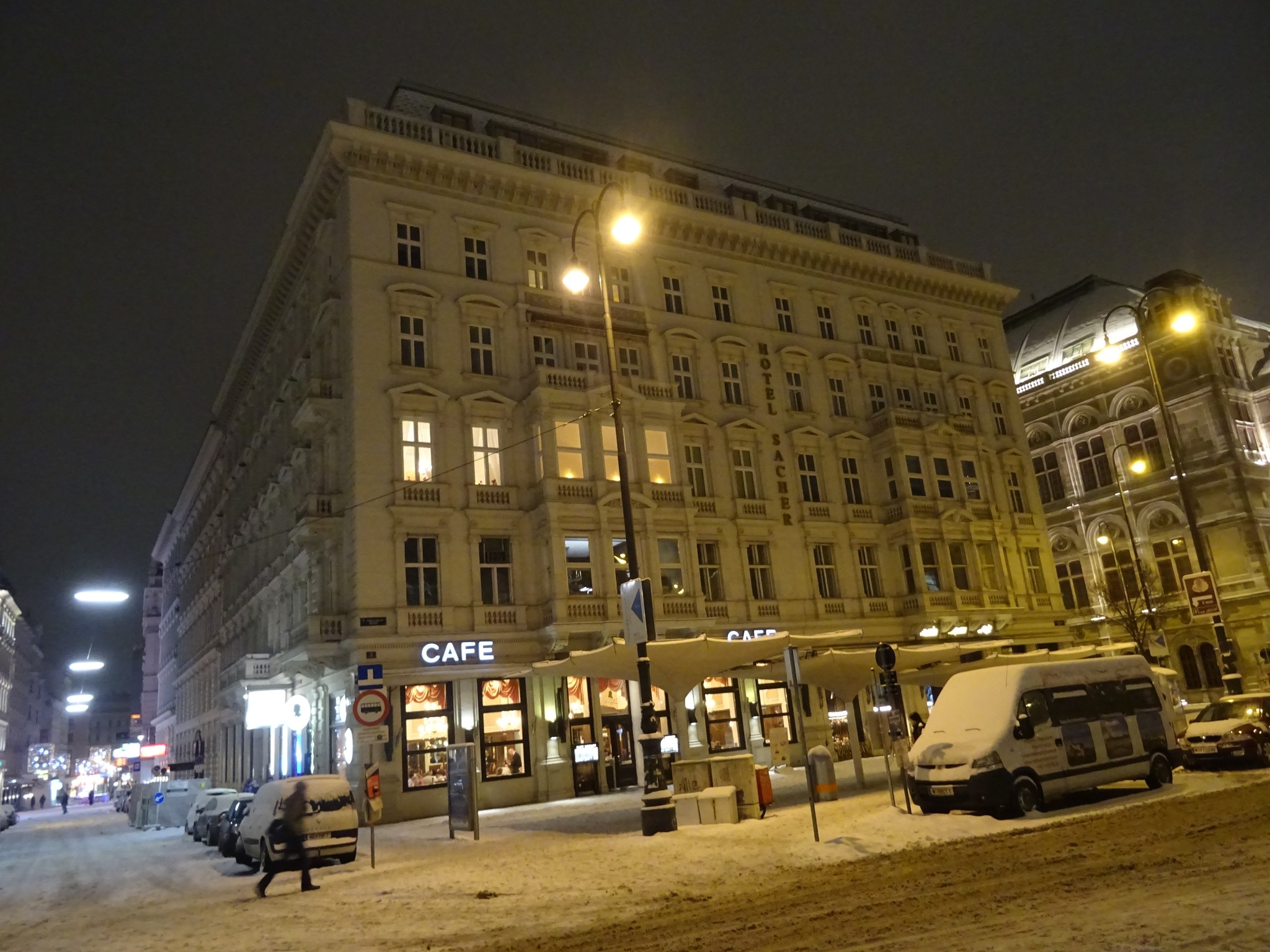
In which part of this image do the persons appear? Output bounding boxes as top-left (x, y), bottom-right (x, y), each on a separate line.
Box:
top-left (61, 792), bottom-right (69, 814)
top-left (508, 748), bottom-right (521, 773)
top-left (39, 794), bottom-right (46, 809)
top-left (408, 767), bottom-right (447, 788)
top-left (243, 779), bottom-right (259, 793)
top-left (711, 740), bottom-right (721, 750)
top-left (30, 796), bottom-right (35, 810)
top-left (909, 711), bottom-right (927, 742)
top-left (485, 750), bottom-right (497, 775)
top-left (251, 781), bottom-right (321, 898)
top-left (88, 790), bottom-right (94, 805)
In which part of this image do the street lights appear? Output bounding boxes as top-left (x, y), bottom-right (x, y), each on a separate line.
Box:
top-left (1096, 520), bottom-right (1149, 662)
top-left (1092, 286), bottom-right (1243, 695)
top-left (1111, 443), bottom-right (1167, 667)
top-left (557, 181), bottom-right (679, 839)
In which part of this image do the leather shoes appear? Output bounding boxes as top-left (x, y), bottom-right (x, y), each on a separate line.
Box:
top-left (301, 884), bottom-right (320, 892)
top-left (253, 886), bottom-right (267, 899)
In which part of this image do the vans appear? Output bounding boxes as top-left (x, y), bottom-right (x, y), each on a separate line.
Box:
top-left (233, 774), bottom-right (359, 875)
top-left (899, 653), bottom-right (1187, 822)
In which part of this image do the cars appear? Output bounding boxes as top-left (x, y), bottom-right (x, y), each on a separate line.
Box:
top-left (183, 787), bottom-right (256, 858)
top-left (1178, 690), bottom-right (1270, 771)
top-left (0, 802), bottom-right (17, 832)
